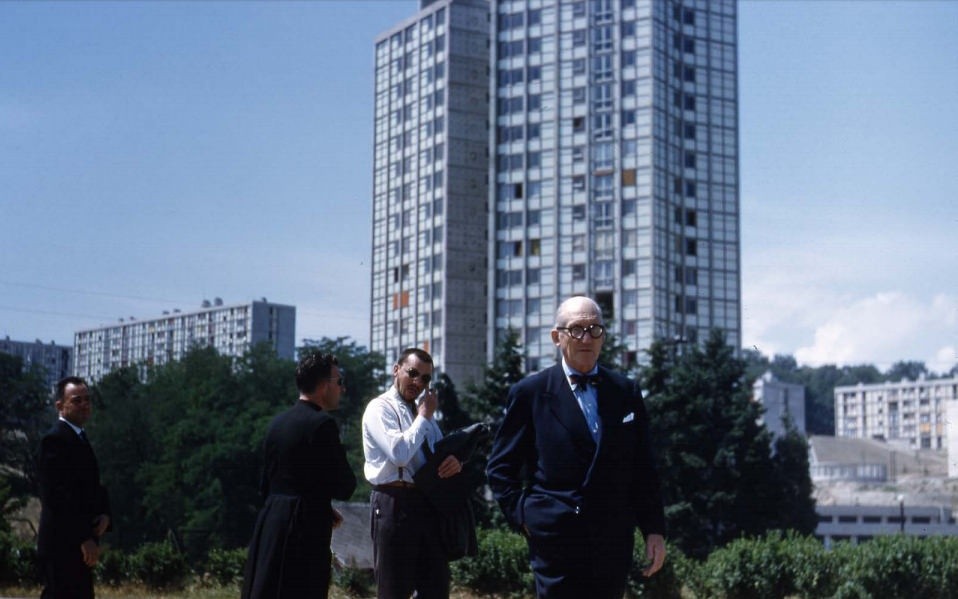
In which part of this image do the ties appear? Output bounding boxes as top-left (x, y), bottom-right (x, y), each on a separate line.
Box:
top-left (78, 430), bottom-right (90, 446)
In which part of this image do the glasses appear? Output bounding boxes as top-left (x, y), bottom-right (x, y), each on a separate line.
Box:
top-left (555, 323), bottom-right (605, 340)
top-left (321, 376), bottom-right (343, 386)
top-left (399, 366), bottom-right (431, 384)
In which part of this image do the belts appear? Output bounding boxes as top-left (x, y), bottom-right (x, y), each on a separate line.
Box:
top-left (386, 481), bottom-right (417, 487)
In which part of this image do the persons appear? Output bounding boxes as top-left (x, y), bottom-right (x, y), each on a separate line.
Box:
top-left (34, 377), bottom-right (114, 599)
top-left (241, 351), bottom-right (359, 599)
top-left (362, 349), bottom-right (462, 599)
top-left (486, 295), bottom-right (668, 599)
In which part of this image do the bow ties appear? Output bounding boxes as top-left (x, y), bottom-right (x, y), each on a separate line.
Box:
top-left (570, 374), bottom-right (604, 393)
